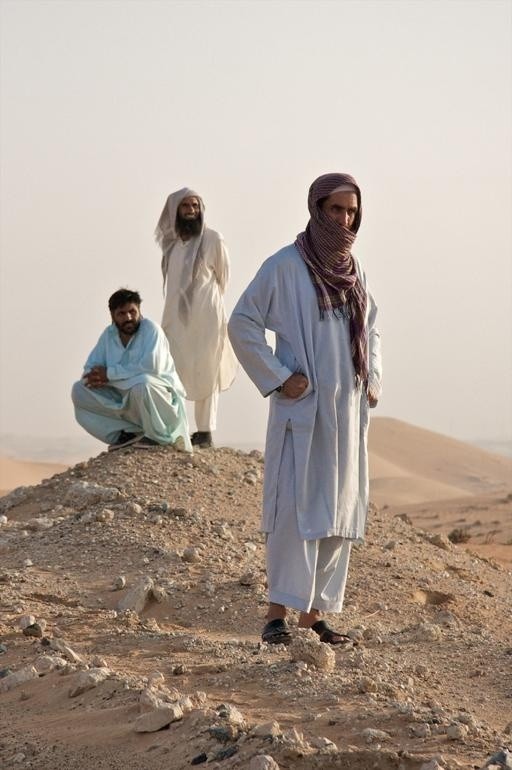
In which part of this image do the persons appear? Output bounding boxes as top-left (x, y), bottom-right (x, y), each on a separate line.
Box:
top-left (71, 286), bottom-right (199, 458)
top-left (150, 187), bottom-right (240, 451)
top-left (228, 172), bottom-right (383, 644)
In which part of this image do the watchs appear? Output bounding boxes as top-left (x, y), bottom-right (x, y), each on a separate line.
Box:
top-left (276, 383), bottom-right (285, 393)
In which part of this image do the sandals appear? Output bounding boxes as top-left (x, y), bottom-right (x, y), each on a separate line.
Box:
top-left (262, 619), bottom-right (292, 645)
top-left (311, 619), bottom-right (353, 644)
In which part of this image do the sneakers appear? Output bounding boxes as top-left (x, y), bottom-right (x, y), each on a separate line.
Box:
top-left (190, 431), bottom-right (213, 449)
top-left (132, 436), bottom-right (159, 449)
top-left (108, 429), bottom-right (145, 452)
